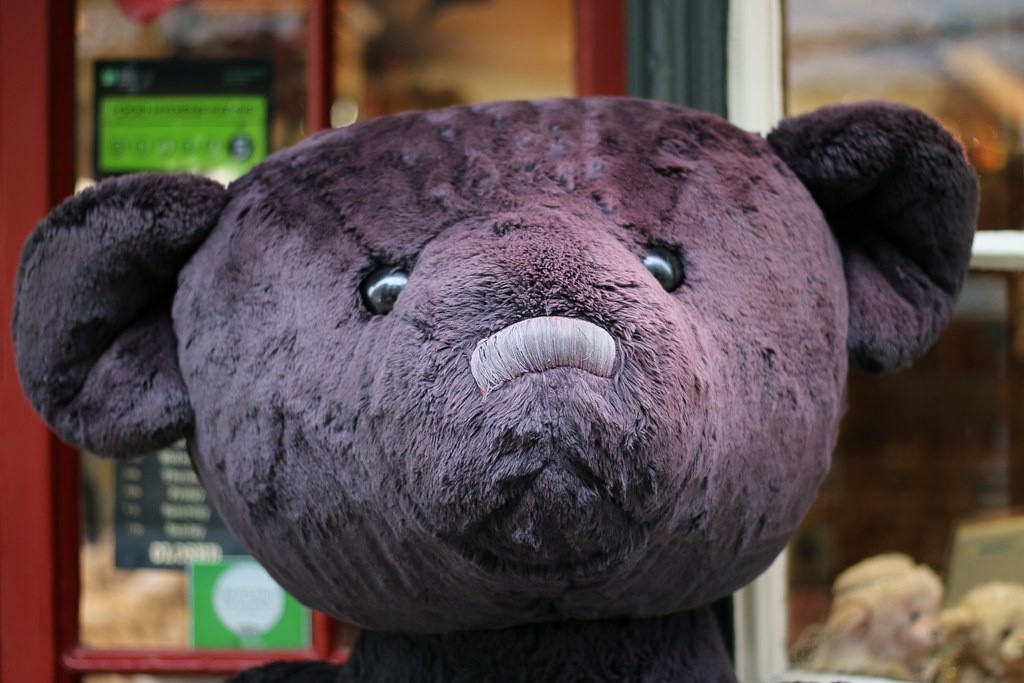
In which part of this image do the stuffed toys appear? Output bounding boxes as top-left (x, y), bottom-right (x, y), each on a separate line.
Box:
top-left (805, 554), bottom-right (1024, 683)
top-left (13, 97), bottom-right (978, 682)
